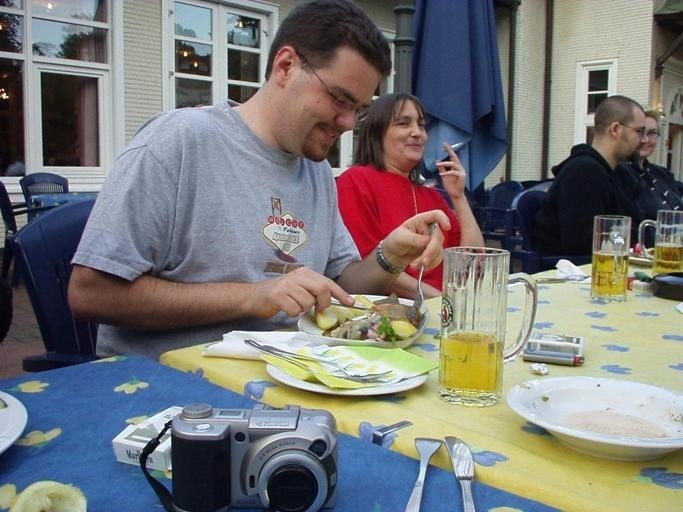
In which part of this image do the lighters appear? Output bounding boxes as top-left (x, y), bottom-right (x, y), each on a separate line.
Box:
top-left (634, 271), bottom-right (652, 283)
top-left (522, 348), bottom-right (585, 366)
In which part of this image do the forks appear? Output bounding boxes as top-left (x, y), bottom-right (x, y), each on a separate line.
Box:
top-left (400, 436), bottom-right (445, 512)
top-left (412, 223), bottom-right (436, 308)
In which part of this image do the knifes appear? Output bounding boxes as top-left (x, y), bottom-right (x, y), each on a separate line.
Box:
top-left (441, 435), bottom-right (478, 510)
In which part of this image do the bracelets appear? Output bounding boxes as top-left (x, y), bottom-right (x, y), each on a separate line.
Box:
top-left (376, 240), bottom-right (408, 275)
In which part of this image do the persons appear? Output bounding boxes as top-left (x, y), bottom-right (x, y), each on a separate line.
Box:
top-left (335, 92), bottom-right (486, 301)
top-left (4, 152), bottom-right (25, 176)
top-left (66, 0), bottom-right (451, 361)
top-left (544, 95), bottom-right (683, 256)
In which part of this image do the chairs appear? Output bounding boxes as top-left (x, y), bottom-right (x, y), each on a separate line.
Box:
top-left (1, 172), bottom-right (98, 294)
top-left (12, 198), bottom-right (110, 368)
top-left (437, 180), bottom-right (549, 268)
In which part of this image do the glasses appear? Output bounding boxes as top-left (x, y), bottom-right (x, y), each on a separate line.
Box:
top-left (294, 47), bottom-right (370, 123)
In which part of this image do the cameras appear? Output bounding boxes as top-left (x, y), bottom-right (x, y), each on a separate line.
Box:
top-left (170, 402), bottom-right (338, 512)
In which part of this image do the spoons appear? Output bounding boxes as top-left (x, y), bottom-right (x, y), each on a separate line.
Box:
top-left (261, 344), bottom-right (393, 381)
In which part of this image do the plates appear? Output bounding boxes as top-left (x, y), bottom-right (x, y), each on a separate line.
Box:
top-left (295, 294), bottom-right (431, 350)
top-left (504, 376), bottom-right (683, 461)
top-left (0, 388), bottom-right (27, 456)
top-left (265, 348), bottom-right (428, 398)
top-left (628, 247), bottom-right (653, 267)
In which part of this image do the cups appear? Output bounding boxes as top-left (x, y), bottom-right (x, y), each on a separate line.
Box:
top-left (437, 247), bottom-right (539, 404)
top-left (637, 210), bottom-right (682, 276)
top-left (588, 215), bottom-right (630, 301)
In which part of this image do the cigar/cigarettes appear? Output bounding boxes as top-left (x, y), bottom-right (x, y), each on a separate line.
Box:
top-left (442, 142), bottom-right (462, 152)
